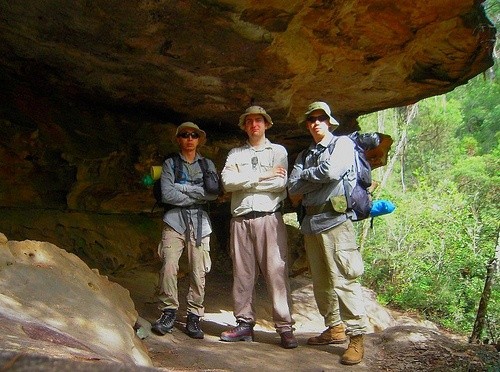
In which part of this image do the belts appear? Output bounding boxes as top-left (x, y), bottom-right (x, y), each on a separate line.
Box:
top-left (240, 211), bottom-right (272, 220)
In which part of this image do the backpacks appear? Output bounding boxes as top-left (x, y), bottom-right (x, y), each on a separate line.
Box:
top-left (302, 131), bottom-right (379, 221)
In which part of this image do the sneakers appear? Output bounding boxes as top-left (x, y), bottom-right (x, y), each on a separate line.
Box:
top-left (280, 332), bottom-right (298, 348)
top-left (341, 335), bottom-right (364, 363)
top-left (150, 313), bottom-right (176, 336)
top-left (220, 321), bottom-right (254, 342)
top-left (183, 318), bottom-right (204, 339)
top-left (307, 325), bottom-right (347, 344)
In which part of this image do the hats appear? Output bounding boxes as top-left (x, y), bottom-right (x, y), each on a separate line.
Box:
top-left (304, 102), bottom-right (339, 132)
top-left (238, 106), bottom-right (273, 130)
top-left (175, 122), bottom-right (206, 151)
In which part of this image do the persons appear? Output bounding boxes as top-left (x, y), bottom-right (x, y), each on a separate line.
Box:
top-left (150, 122), bottom-right (219, 339)
top-left (288, 101), bottom-right (366, 364)
top-left (219, 106), bottom-right (300, 349)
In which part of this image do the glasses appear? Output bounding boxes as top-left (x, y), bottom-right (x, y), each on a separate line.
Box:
top-left (177, 132), bottom-right (199, 139)
top-left (306, 115), bottom-right (329, 122)
